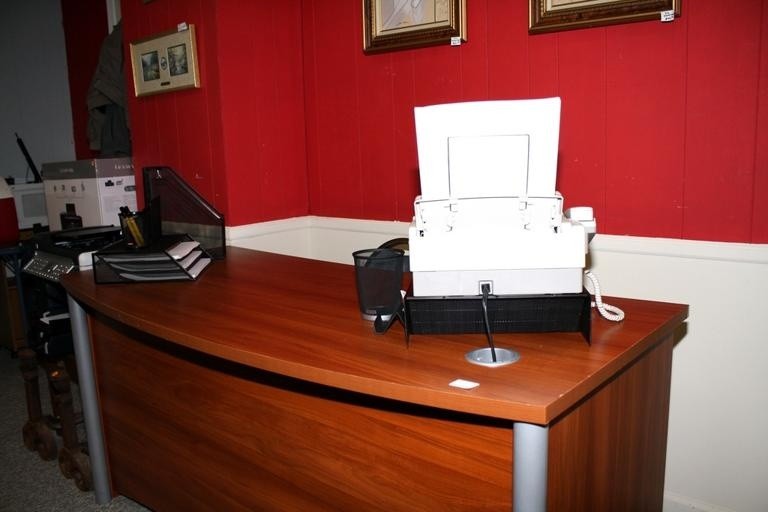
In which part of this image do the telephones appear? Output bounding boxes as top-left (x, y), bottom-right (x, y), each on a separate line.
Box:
top-left (565, 207), bottom-right (596, 243)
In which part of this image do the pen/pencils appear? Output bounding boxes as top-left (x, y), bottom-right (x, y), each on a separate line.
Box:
top-left (119, 206), bottom-right (144, 247)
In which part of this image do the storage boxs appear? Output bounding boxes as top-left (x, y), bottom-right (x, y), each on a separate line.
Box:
top-left (40, 155), bottom-right (139, 231)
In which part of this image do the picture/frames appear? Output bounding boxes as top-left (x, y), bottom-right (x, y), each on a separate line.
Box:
top-left (360, 1), bottom-right (468, 57)
top-left (128, 22), bottom-right (202, 102)
top-left (525, 0), bottom-right (683, 37)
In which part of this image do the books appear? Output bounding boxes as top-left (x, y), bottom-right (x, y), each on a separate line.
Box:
top-left (102, 240), bottom-right (212, 281)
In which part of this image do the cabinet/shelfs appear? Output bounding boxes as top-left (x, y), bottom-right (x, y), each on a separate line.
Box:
top-left (0, 256), bottom-right (27, 355)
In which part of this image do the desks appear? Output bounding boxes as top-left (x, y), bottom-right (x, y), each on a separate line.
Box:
top-left (54, 243), bottom-right (690, 512)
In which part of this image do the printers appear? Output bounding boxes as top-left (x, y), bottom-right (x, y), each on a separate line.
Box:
top-left (59, 203), bottom-right (83, 230)
top-left (406, 95), bottom-right (590, 297)
